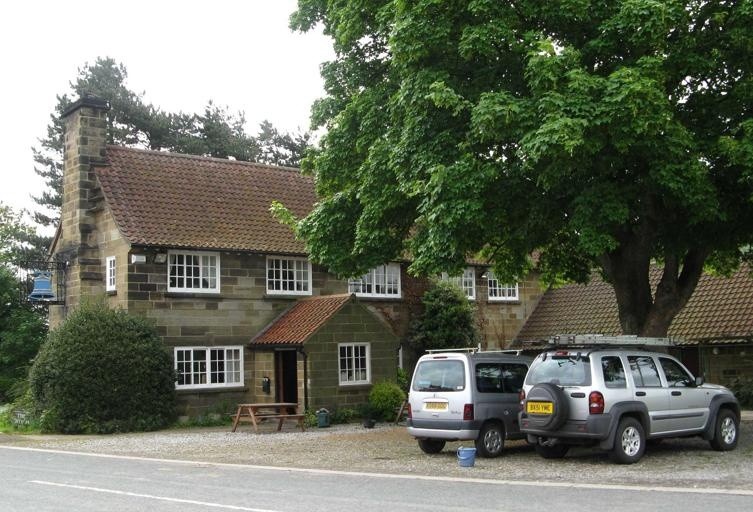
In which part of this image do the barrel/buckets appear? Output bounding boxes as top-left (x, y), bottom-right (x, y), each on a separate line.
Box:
top-left (457, 447), bottom-right (477, 467)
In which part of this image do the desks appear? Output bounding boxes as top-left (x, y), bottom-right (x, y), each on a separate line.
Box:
top-left (232, 403), bottom-right (299, 433)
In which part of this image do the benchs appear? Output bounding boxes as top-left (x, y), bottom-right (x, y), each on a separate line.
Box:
top-left (229, 413), bottom-right (284, 428)
top-left (254, 414), bottom-right (307, 433)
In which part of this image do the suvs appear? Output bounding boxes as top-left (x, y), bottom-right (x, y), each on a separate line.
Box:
top-left (517, 332), bottom-right (741, 465)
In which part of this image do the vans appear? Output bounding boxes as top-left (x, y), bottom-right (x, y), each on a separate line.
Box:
top-left (401, 344), bottom-right (530, 458)
